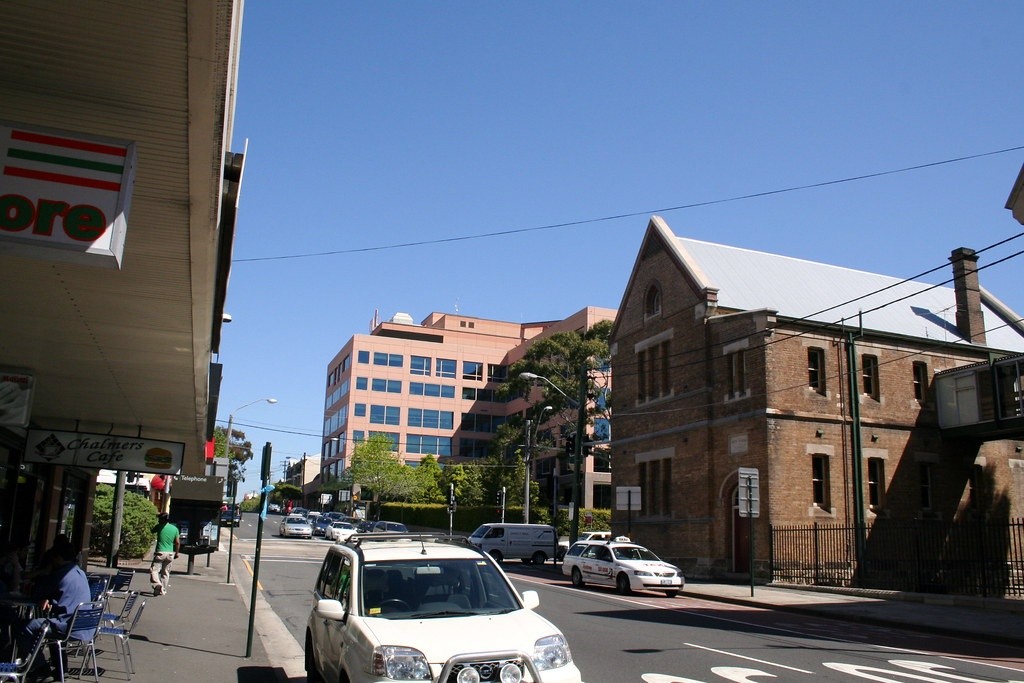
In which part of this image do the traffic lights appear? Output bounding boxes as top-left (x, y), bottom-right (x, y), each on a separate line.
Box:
top-left (497, 489), bottom-right (505, 507)
top-left (450, 501), bottom-right (457, 513)
top-left (496, 509), bottom-right (502, 515)
top-left (447, 488), bottom-right (452, 498)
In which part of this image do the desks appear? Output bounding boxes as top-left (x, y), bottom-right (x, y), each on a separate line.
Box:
top-left (0, 596), bottom-right (44, 662)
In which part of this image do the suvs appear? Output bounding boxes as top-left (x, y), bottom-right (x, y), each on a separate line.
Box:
top-left (556, 531), bottom-right (611, 561)
top-left (304, 532), bottom-right (582, 683)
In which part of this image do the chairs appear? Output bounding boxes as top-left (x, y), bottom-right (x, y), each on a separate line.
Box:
top-left (86, 567), bottom-right (135, 625)
top-left (0, 620), bottom-right (50, 683)
top-left (78, 600), bottom-right (146, 680)
top-left (365, 569), bottom-right (402, 608)
top-left (40, 601), bottom-right (107, 683)
top-left (74, 590), bottom-right (142, 660)
top-left (424, 571), bottom-right (471, 609)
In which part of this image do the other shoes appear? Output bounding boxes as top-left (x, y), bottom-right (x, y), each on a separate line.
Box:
top-left (154, 585), bottom-right (161, 596)
top-left (51, 668), bottom-right (69, 678)
top-left (160, 592), bottom-right (165, 595)
top-left (32, 673), bottom-right (54, 683)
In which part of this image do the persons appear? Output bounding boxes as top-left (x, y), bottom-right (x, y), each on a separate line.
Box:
top-left (149, 513), bottom-right (180, 597)
top-left (0, 534), bottom-right (91, 683)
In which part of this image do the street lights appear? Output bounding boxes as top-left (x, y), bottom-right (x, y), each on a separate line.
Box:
top-left (525, 406), bottom-right (553, 523)
top-left (520, 372), bottom-right (586, 545)
top-left (224, 398), bottom-right (277, 458)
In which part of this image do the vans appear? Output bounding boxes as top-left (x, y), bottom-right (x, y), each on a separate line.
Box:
top-left (466, 523), bottom-right (558, 566)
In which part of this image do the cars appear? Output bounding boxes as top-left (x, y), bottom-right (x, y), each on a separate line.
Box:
top-left (279, 512), bottom-right (310, 540)
top-left (293, 506), bottom-right (309, 518)
top-left (561, 536), bottom-right (686, 598)
top-left (221, 509), bottom-right (242, 526)
top-left (307, 511), bottom-right (412, 548)
